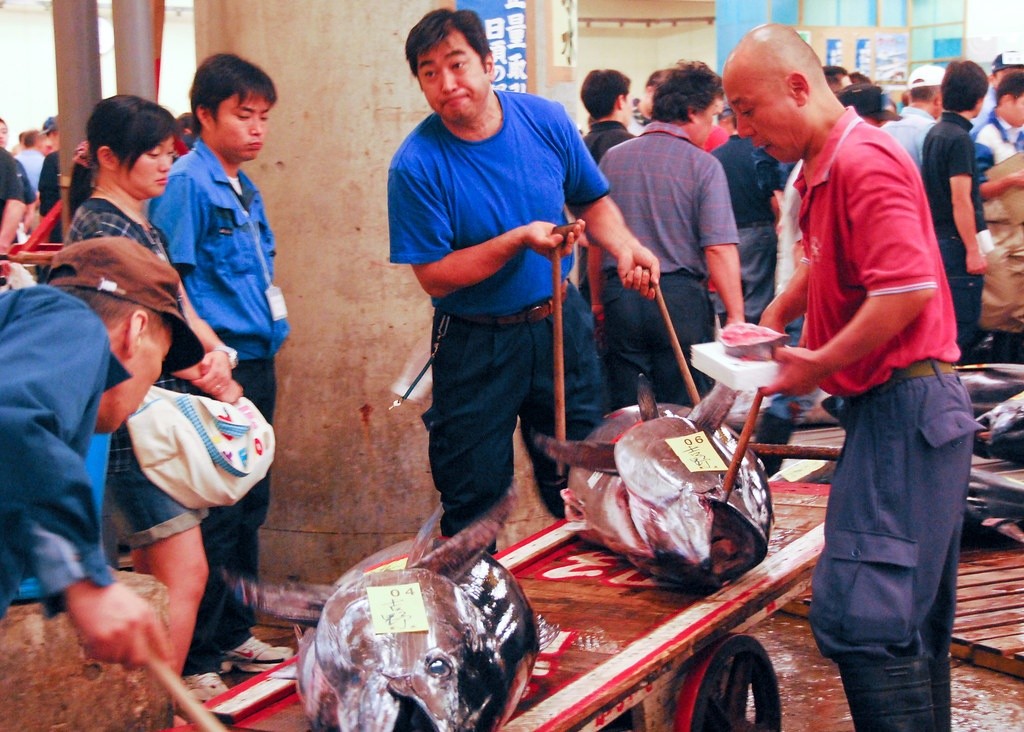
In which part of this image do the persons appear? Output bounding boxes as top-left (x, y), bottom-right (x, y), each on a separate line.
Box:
top-left (386, 9), bottom-right (662, 544)
top-left (0, 53), bottom-right (294, 728)
top-left (578, 49), bottom-right (1024, 409)
top-left (722, 25), bottom-right (975, 732)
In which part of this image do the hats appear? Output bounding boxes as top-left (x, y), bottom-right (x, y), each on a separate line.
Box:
top-left (906, 66), bottom-right (945, 89)
top-left (838, 82), bottom-right (903, 121)
top-left (37, 117), bottom-right (57, 136)
top-left (991, 51), bottom-right (1024, 73)
top-left (48, 236), bottom-right (206, 376)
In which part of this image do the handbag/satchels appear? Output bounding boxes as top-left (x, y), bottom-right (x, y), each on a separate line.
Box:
top-left (124, 387), bottom-right (278, 509)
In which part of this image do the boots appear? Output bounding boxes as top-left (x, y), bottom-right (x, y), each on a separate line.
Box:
top-left (841, 654), bottom-right (950, 732)
top-left (755, 408), bottom-right (793, 476)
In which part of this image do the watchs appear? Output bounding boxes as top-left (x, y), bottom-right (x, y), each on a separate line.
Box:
top-left (212, 345), bottom-right (239, 369)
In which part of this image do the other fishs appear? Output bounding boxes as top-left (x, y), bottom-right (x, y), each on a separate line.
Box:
top-left (723, 362), bottom-right (1024, 549)
top-left (715, 318), bottom-right (790, 362)
top-left (293, 463), bottom-right (560, 731)
top-left (528, 370), bottom-right (776, 585)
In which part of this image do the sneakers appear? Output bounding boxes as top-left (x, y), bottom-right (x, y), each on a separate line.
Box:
top-left (219, 636), bottom-right (294, 673)
top-left (181, 672), bottom-right (228, 704)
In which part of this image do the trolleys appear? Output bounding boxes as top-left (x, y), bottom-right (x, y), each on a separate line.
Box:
top-left (170, 437), bottom-right (842, 732)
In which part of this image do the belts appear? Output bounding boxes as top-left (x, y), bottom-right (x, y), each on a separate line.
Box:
top-left (455, 277), bottom-right (569, 325)
top-left (874, 359), bottom-right (955, 394)
top-left (736, 220), bottom-right (772, 228)
